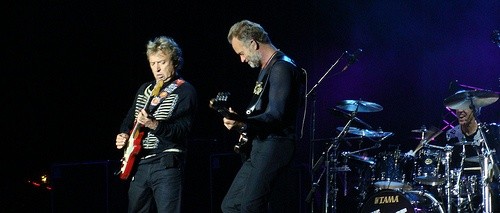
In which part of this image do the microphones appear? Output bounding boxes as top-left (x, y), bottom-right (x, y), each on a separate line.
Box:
top-left (343, 48), bottom-right (364, 72)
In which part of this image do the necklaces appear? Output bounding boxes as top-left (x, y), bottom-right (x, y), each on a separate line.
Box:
top-left (256, 48), bottom-right (280, 81)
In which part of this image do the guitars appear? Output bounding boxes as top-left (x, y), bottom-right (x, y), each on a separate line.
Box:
top-left (115, 79), bottom-right (164, 180)
top-left (209, 92), bottom-right (255, 148)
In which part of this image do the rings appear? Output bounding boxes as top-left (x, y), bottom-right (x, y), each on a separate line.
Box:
top-left (141, 117), bottom-right (145, 122)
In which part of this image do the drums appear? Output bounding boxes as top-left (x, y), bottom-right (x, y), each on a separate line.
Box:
top-left (343, 154), bottom-right (379, 172)
top-left (337, 126), bottom-right (380, 138)
top-left (358, 189), bottom-right (444, 213)
top-left (337, 99), bottom-right (383, 115)
top-left (371, 179), bottom-right (411, 194)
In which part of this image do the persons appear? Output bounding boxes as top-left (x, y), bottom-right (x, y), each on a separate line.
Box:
top-left (445, 100), bottom-right (500, 151)
top-left (115, 35), bottom-right (198, 213)
top-left (220, 19), bottom-right (305, 213)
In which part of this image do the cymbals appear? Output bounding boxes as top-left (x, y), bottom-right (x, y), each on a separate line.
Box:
top-left (443, 89), bottom-right (499, 110)
